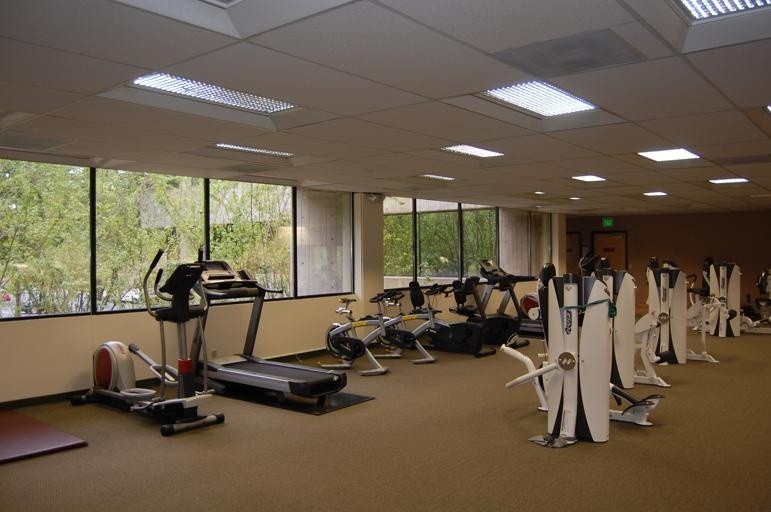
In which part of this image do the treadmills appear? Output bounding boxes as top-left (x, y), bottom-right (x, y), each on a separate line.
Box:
top-left (193, 260), bottom-right (348, 406)
top-left (466, 258), bottom-right (545, 337)
